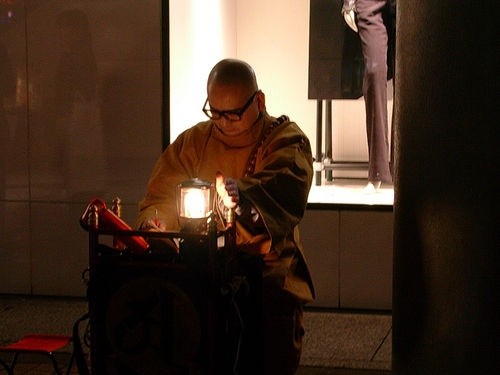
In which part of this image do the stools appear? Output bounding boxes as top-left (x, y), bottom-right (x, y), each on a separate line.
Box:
top-left (0, 333), bottom-right (76, 375)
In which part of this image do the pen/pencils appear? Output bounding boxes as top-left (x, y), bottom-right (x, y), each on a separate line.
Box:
top-left (154, 208), bottom-right (160, 229)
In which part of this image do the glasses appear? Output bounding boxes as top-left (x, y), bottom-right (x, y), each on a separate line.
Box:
top-left (202, 89), bottom-right (258, 121)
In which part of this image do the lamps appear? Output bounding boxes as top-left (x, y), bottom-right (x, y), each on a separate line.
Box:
top-left (177, 177), bottom-right (215, 231)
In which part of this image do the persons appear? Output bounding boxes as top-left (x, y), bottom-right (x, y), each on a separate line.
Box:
top-left (342, 1), bottom-right (395, 189)
top-left (135, 57), bottom-right (315, 374)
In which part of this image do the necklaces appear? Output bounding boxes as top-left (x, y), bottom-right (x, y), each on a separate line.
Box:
top-left (239, 115), bottom-right (291, 184)
